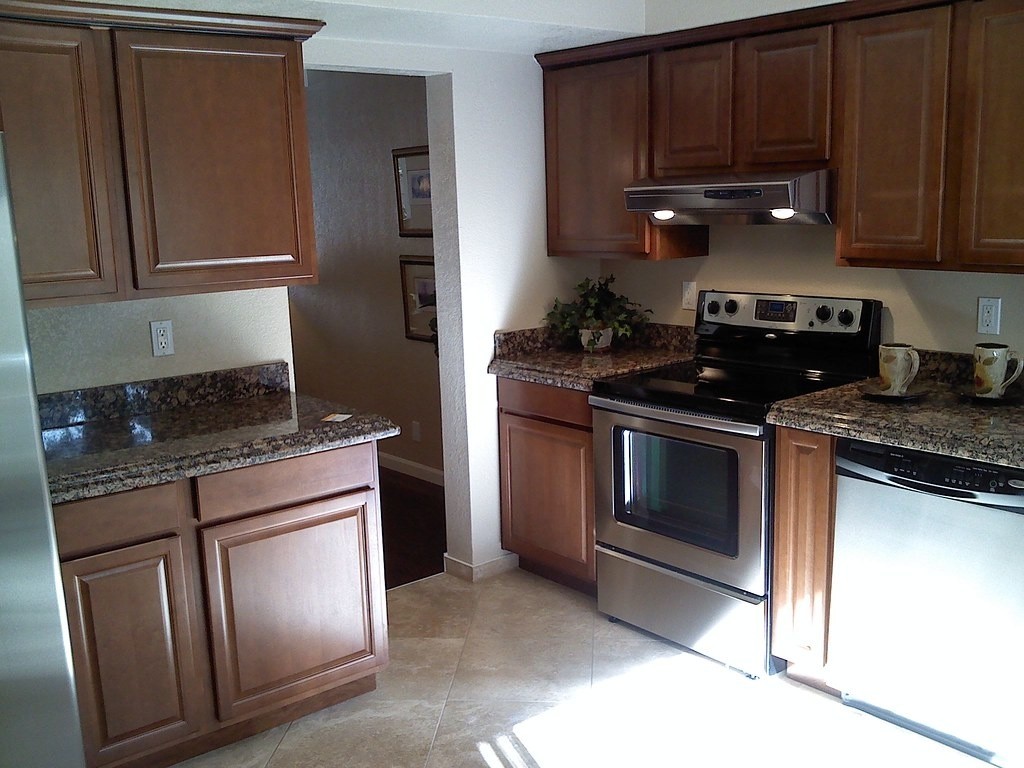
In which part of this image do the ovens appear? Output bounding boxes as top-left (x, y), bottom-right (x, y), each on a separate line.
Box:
top-left (591, 407), bottom-right (787, 679)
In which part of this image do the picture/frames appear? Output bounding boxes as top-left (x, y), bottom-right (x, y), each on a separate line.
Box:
top-left (398, 255), bottom-right (439, 343)
top-left (391, 146), bottom-right (432, 238)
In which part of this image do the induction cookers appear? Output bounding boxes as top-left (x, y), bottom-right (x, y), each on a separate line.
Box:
top-left (589, 289), bottom-right (882, 423)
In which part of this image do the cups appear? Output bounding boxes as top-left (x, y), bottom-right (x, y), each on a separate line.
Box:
top-left (879, 343), bottom-right (920, 396)
top-left (973, 343), bottom-right (1024, 398)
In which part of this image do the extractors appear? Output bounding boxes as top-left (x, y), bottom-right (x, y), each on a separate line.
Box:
top-left (623, 167), bottom-right (835, 227)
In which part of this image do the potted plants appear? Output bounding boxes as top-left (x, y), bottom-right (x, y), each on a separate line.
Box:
top-left (547, 273), bottom-right (640, 349)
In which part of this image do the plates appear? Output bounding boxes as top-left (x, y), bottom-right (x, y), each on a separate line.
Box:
top-left (857, 385), bottom-right (932, 399)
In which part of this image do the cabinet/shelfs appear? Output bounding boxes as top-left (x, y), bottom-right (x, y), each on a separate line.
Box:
top-left (1, 0), bottom-right (118, 308)
top-left (960, 0), bottom-right (1023, 274)
top-left (650, 25), bottom-right (835, 178)
top-left (835, 7), bottom-right (954, 271)
top-left (195, 441), bottom-right (393, 731)
top-left (496, 374), bottom-right (599, 596)
top-left (47, 482), bottom-right (220, 767)
top-left (535, 36), bottom-right (712, 261)
top-left (115, 3), bottom-right (327, 298)
top-left (771, 428), bottom-right (833, 667)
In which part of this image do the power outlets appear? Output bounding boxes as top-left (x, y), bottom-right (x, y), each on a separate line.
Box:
top-left (149, 319), bottom-right (175, 356)
top-left (683, 281), bottom-right (699, 310)
top-left (974, 298), bottom-right (1001, 333)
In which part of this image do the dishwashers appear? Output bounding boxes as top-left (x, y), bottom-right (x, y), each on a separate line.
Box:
top-left (828, 438), bottom-right (1023, 760)
